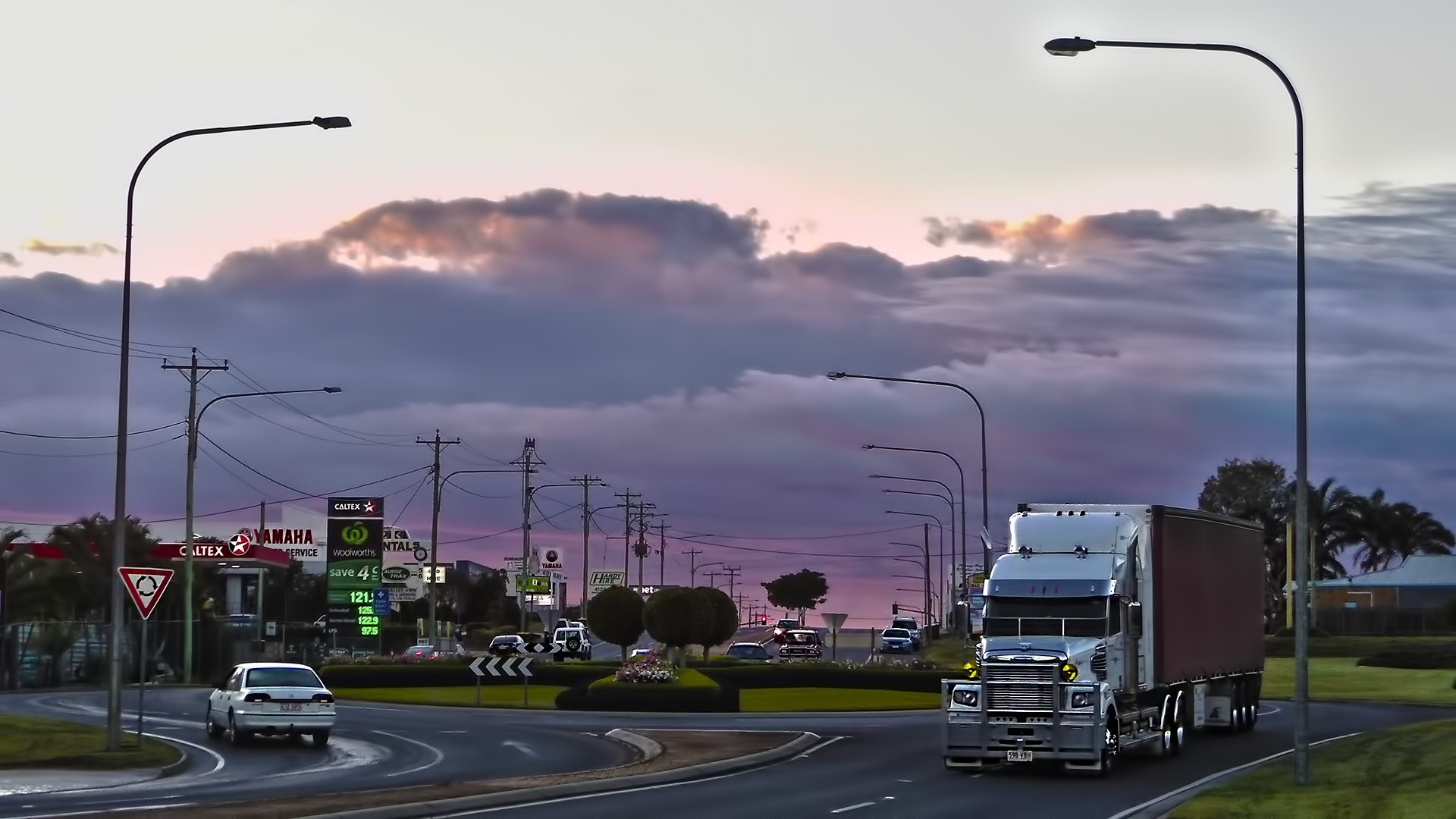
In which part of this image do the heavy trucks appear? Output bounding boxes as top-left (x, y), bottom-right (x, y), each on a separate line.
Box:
top-left (942, 503), bottom-right (1264, 769)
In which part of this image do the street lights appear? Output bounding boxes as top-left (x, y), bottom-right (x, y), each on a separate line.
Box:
top-left (692, 562), bottom-right (723, 588)
top-left (583, 503), bottom-right (653, 620)
top-left (827, 371), bottom-right (991, 635)
top-left (185, 388), bottom-right (342, 688)
top-left (521, 482), bottom-right (610, 631)
top-left (1044, 36), bottom-right (1310, 789)
top-left (428, 469), bottom-right (538, 647)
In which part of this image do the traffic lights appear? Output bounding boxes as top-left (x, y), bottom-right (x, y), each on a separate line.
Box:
top-left (761, 617), bottom-right (766, 625)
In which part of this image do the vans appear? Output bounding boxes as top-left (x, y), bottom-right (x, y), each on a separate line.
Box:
top-left (225, 614), bottom-right (257, 626)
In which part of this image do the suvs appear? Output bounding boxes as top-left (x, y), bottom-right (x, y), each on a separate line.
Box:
top-left (553, 622), bottom-right (592, 661)
top-left (720, 641), bottom-right (774, 663)
top-left (892, 615), bottom-right (922, 651)
top-left (779, 627), bottom-right (824, 664)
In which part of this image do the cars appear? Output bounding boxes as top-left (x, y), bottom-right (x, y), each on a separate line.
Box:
top-left (489, 636), bottom-right (524, 656)
top-left (772, 619), bottom-right (803, 641)
top-left (402, 645), bottom-right (465, 663)
top-left (206, 663), bottom-right (337, 749)
top-left (880, 629), bottom-right (912, 654)
top-left (314, 615), bottom-right (326, 633)
